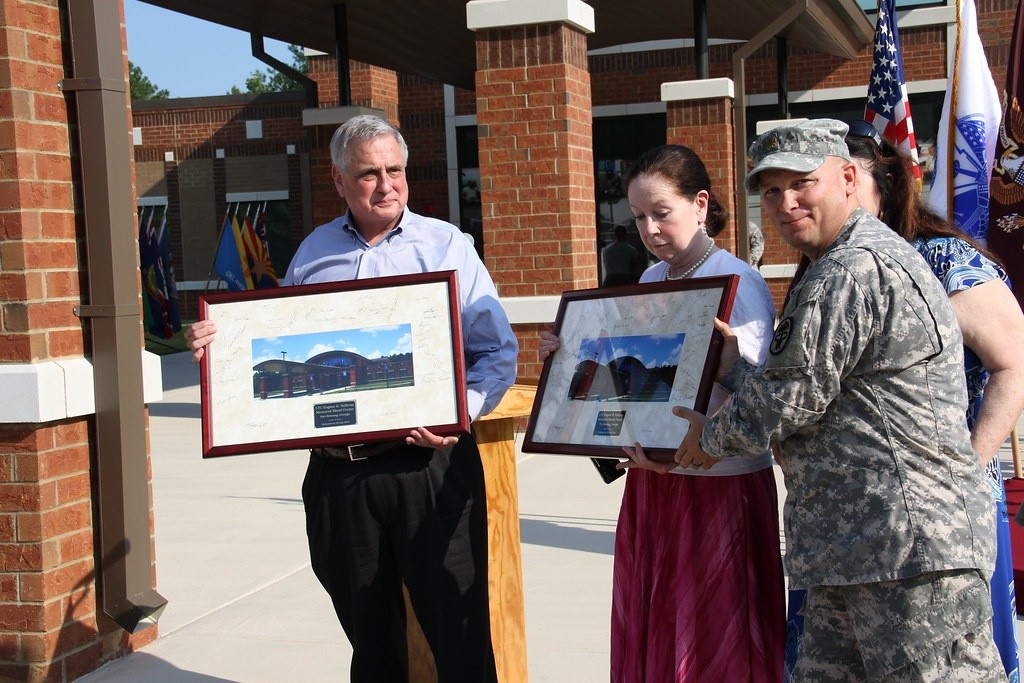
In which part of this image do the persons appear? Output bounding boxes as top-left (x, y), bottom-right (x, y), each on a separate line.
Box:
top-left (782, 119), bottom-right (1024, 683)
top-left (748, 222), bottom-right (765, 271)
top-left (600, 225), bottom-right (640, 287)
top-left (537, 146), bottom-right (787, 683)
top-left (183, 115), bottom-right (520, 683)
top-left (673, 119), bottom-right (1009, 683)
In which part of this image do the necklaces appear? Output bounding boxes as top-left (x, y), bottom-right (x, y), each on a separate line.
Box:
top-left (666, 236), bottom-right (716, 280)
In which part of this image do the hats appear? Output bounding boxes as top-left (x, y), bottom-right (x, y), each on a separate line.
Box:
top-left (743, 118), bottom-right (852, 195)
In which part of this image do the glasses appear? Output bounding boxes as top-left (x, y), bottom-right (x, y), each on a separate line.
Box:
top-left (845, 118), bottom-right (886, 156)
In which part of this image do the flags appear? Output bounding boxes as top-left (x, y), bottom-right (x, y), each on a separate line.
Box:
top-left (929, 0), bottom-right (1003, 251)
top-left (139, 213), bottom-right (182, 340)
top-left (213, 216), bottom-right (281, 292)
top-left (862, 0), bottom-right (922, 196)
top-left (986, 0), bottom-right (1024, 312)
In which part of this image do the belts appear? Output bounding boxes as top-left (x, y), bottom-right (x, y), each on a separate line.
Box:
top-left (312, 440), bottom-right (401, 462)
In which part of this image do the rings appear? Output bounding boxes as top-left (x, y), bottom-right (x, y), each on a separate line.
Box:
top-left (691, 460), bottom-right (701, 467)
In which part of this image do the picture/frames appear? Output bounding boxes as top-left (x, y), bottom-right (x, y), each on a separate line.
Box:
top-left (197, 268), bottom-right (471, 460)
top-left (520, 272), bottom-right (740, 463)
